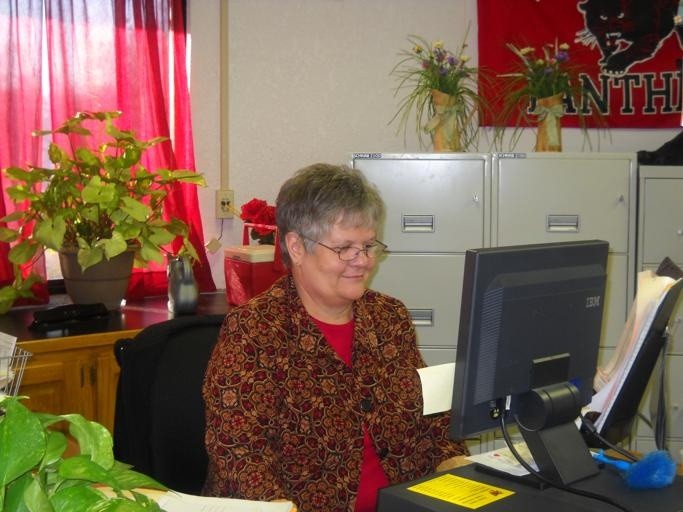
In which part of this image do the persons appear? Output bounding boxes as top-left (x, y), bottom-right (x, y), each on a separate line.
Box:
top-left (201, 162), bottom-right (469, 512)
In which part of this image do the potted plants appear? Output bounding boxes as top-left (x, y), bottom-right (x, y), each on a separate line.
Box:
top-left (0, 110), bottom-right (208, 315)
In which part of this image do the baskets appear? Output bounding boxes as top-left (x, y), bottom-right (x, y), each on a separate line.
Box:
top-left (0, 346), bottom-right (34, 415)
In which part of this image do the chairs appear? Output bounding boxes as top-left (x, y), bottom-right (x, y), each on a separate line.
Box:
top-left (113, 314), bottom-right (234, 495)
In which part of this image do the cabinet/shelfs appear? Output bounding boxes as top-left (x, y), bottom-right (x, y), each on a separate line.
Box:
top-left (490, 153), bottom-right (637, 369)
top-left (347, 152), bottom-right (489, 366)
top-left (631, 165), bottom-right (683, 468)
top-left (8, 329), bottom-right (145, 474)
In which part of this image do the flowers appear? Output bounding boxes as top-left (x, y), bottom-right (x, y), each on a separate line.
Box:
top-left (490, 32), bottom-right (613, 153)
top-left (385, 19), bottom-right (496, 153)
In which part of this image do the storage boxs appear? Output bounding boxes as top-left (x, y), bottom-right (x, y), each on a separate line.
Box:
top-left (223, 221), bottom-right (282, 307)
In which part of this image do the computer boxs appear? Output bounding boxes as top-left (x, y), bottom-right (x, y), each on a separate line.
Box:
top-left (375, 448), bottom-right (683, 512)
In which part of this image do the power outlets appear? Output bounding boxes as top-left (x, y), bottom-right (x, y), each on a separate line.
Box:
top-left (216, 190), bottom-right (233, 219)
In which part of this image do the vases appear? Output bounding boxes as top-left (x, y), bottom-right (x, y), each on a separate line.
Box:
top-left (534, 93), bottom-right (562, 153)
top-left (423, 88), bottom-right (460, 153)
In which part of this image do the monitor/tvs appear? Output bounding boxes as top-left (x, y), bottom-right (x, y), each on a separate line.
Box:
top-left (448, 240), bottom-right (609, 491)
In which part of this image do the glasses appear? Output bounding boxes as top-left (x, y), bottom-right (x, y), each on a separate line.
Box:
top-left (299, 232), bottom-right (388, 262)
